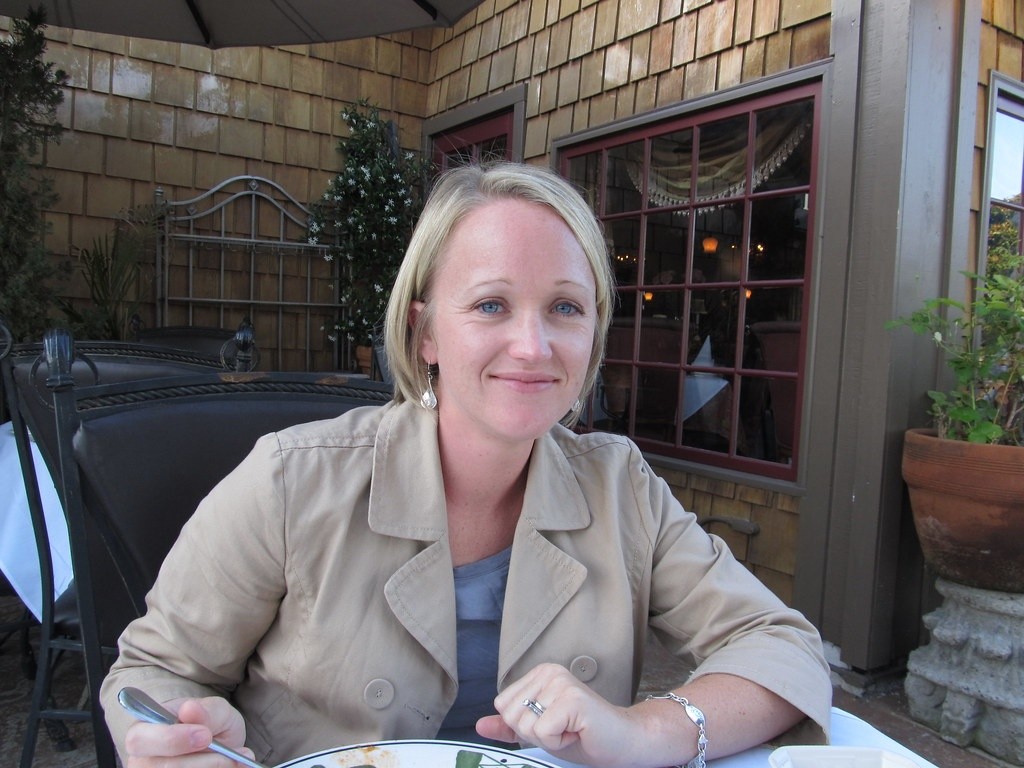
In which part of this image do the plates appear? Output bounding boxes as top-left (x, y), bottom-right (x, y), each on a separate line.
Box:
top-left (279, 740), bottom-right (553, 768)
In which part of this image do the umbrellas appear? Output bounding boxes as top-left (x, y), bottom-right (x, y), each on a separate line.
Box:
top-left (0, 0), bottom-right (486, 52)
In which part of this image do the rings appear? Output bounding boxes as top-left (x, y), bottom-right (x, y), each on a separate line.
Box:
top-left (521, 697), bottom-right (546, 715)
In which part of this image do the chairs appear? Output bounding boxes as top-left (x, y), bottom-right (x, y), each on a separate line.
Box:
top-left (0, 320), bottom-right (264, 768)
top-left (600, 318), bottom-right (696, 440)
top-left (43, 325), bottom-right (396, 768)
top-left (751, 321), bottom-right (801, 457)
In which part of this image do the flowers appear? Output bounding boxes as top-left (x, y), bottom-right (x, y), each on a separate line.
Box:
top-left (305, 98), bottom-right (440, 344)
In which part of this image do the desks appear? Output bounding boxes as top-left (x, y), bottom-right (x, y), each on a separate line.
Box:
top-left (507, 686), bottom-right (1005, 768)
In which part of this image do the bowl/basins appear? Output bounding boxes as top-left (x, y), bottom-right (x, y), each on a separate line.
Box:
top-left (768, 746), bottom-right (920, 768)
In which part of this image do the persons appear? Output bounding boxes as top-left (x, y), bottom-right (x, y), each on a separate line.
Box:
top-left (96, 163), bottom-right (833, 768)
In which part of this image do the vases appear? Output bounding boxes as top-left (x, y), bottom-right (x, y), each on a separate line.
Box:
top-left (356, 347), bottom-right (387, 375)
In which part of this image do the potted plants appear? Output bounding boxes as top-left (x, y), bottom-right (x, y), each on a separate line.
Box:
top-left (884, 207), bottom-right (1024, 593)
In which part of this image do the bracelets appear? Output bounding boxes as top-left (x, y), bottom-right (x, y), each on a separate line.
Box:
top-left (645, 693), bottom-right (709, 768)
